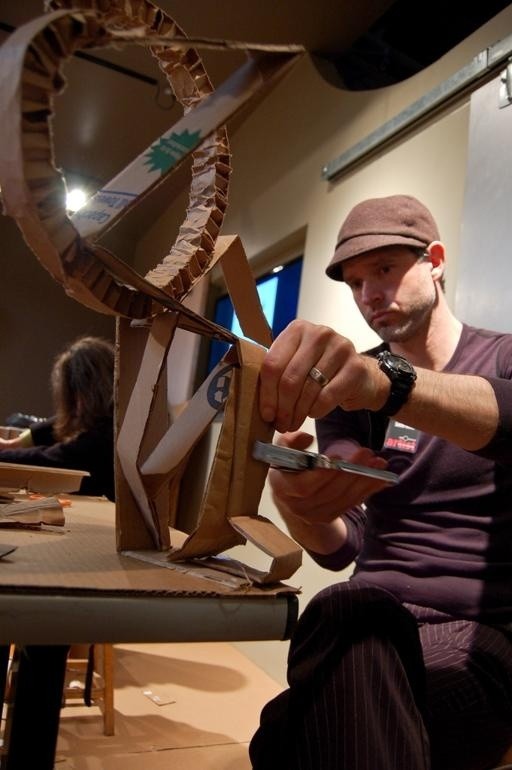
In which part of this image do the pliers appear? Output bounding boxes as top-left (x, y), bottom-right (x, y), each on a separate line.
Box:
top-left (251, 439), bottom-right (400, 484)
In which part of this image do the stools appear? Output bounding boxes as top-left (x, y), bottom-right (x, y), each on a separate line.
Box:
top-left (3, 641), bottom-right (121, 737)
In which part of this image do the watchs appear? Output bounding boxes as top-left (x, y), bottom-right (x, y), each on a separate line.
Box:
top-left (369, 351), bottom-right (418, 420)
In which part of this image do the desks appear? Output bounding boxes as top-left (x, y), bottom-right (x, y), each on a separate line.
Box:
top-left (0, 495), bottom-right (302, 770)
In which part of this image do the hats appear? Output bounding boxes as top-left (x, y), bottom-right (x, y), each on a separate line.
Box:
top-left (326, 196), bottom-right (439, 281)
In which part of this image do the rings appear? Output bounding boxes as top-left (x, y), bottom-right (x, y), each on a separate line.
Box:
top-left (309, 367), bottom-right (330, 388)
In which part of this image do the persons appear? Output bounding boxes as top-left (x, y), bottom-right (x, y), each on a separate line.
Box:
top-left (1, 336), bottom-right (114, 506)
top-left (245, 191), bottom-right (512, 770)
